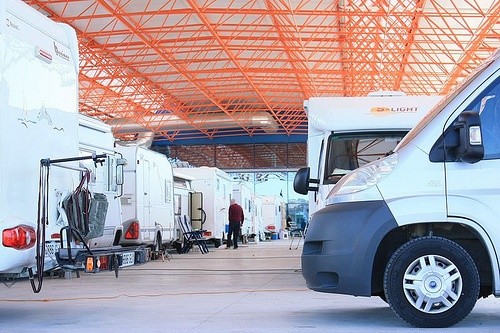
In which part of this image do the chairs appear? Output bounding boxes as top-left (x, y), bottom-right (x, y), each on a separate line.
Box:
top-left (178, 216), bottom-right (204, 254)
top-left (184, 215), bottom-right (210, 253)
top-left (289, 222), bottom-right (309, 250)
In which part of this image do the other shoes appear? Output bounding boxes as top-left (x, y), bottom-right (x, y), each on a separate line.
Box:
top-left (225, 244), bottom-right (239, 249)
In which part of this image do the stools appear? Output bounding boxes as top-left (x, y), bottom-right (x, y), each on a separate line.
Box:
top-left (281, 232), bottom-right (288, 239)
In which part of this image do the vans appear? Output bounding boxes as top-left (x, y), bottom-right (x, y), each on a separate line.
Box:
top-left (300, 53), bottom-right (496, 327)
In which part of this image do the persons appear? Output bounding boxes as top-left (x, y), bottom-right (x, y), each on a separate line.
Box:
top-left (225, 198), bottom-right (245, 249)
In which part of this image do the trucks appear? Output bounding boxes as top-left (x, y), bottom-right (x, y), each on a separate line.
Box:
top-left (0, 1), bottom-right (122, 281)
top-left (293, 92), bottom-right (443, 219)
top-left (77, 114), bottom-right (287, 251)
